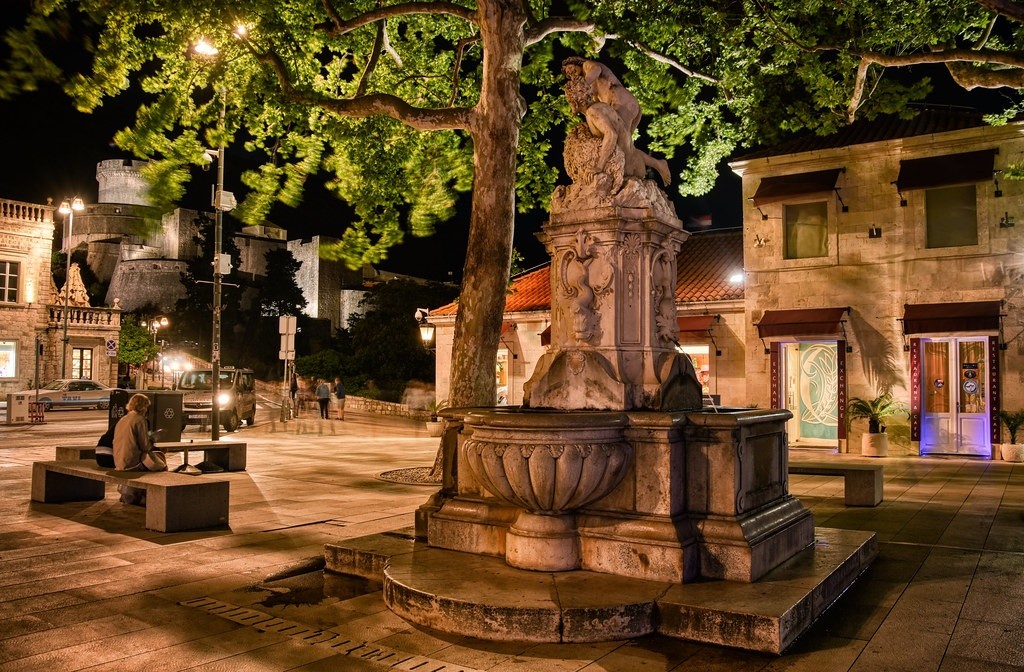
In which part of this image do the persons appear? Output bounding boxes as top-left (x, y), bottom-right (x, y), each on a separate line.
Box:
top-left (315, 380), bottom-right (332, 420)
top-left (563, 57), bottom-right (672, 187)
top-left (95, 393), bottom-right (168, 506)
top-left (333, 377), bottom-right (346, 421)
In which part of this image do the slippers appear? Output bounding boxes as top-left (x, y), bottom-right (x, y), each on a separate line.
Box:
top-left (173, 463), bottom-right (202, 476)
top-left (195, 460), bottom-right (225, 473)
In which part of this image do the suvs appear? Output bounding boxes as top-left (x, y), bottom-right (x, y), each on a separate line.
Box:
top-left (176, 367), bottom-right (257, 432)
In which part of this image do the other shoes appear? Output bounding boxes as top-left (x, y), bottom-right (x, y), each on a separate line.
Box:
top-left (119, 492), bottom-right (143, 505)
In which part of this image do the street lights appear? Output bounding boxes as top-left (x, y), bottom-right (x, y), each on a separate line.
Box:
top-left (191, 19), bottom-right (248, 440)
top-left (57, 192), bottom-right (85, 378)
top-left (149, 315), bottom-right (168, 382)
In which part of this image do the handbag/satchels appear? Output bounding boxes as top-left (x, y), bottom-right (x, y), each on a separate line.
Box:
top-left (140, 445), bottom-right (166, 470)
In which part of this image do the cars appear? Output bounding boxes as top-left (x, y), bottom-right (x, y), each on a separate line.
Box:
top-left (16, 379), bottom-right (122, 411)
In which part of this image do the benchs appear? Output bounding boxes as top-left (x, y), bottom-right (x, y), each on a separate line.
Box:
top-left (56, 440), bottom-right (248, 471)
top-left (31, 459), bottom-right (230, 533)
top-left (787, 458), bottom-right (884, 507)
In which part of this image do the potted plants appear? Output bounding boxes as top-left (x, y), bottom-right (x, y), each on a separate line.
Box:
top-left (998, 410), bottom-right (1024, 462)
top-left (845, 392), bottom-right (911, 457)
top-left (423, 397), bottom-right (449, 437)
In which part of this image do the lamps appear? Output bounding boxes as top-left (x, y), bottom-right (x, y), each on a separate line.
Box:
top-left (419, 322), bottom-right (436, 355)
